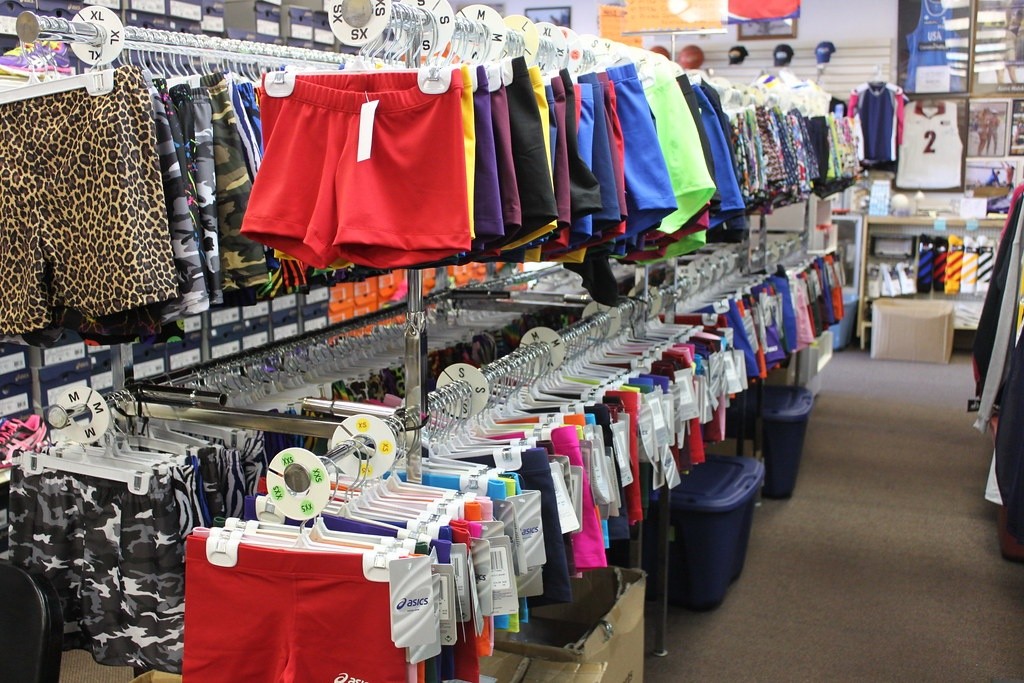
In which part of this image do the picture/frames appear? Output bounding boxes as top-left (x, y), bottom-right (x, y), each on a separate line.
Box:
top-left (737, 18), bottom-right (797, 40)
top-left (524, 6), bottom-right (570, 28)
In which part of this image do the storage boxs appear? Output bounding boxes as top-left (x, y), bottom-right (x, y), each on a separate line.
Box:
top-left (478, 648), bottom-right (609, 683)
top-left (728, 384), bottom-right (814, 500)
top-left (828, 293), bottom-right (858, 350)
top-left (871, 297), bottom-right (955, 366)
top-left (497, 566), bottom-right (650, 683)
top-left (605, 453), bottom-right (765, 616)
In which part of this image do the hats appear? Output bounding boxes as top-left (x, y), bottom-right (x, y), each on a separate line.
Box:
top-left (815, 41), bottom-right (835, 64)
top-left (728, 46), bottom-right (749, 65)
top-left (773, 44), bottom-right (794, 66)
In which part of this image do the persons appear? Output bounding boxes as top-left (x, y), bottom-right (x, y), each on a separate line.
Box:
top-left (977, 108), bottom-right (1000, 154)
top-left (994, 7), bottom-right (1023, 84)
top-left (985, 162), bottom-right (1014, 186)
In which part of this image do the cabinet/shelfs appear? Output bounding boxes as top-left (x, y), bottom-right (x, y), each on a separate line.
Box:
top-left (856, 218), bottom-right (1005, 351)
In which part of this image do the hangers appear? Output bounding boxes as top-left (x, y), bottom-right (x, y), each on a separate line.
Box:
top-left (0, 4), bottom-right (820, 584)
